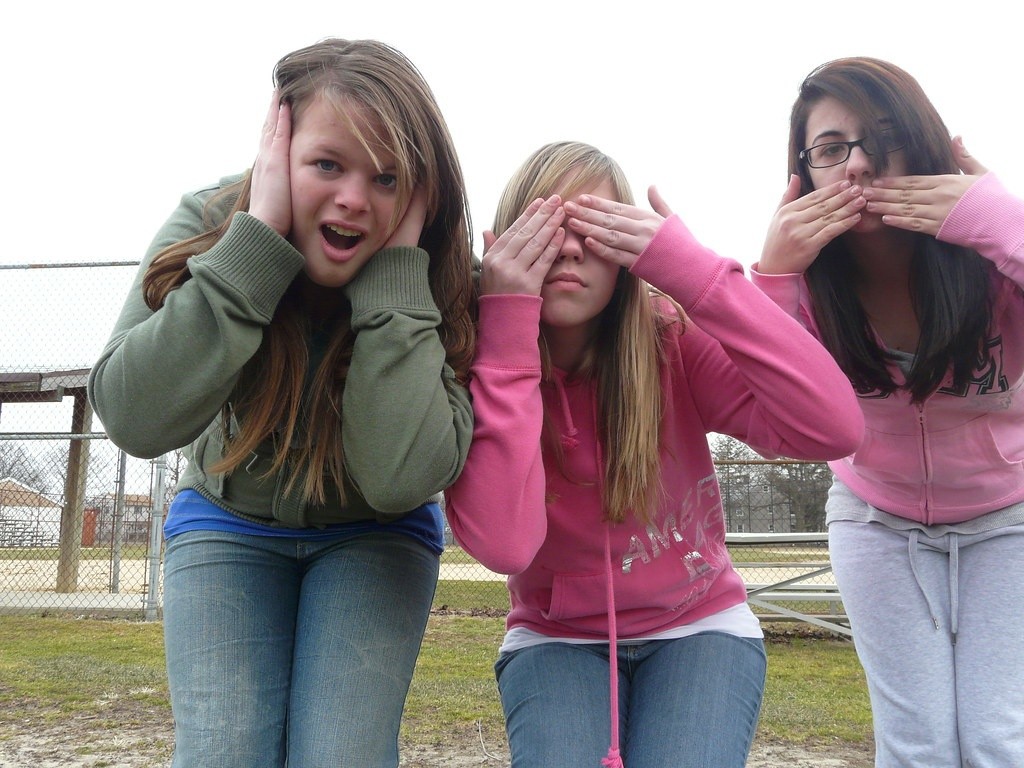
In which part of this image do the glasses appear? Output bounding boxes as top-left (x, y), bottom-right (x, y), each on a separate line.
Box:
top-left (799, 125), bottom-right (908, 169)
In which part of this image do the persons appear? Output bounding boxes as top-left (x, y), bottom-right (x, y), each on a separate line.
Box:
top-left (86, 37), bottom-right (475, 768)
top-left (749, 55), bottom-right (1024, 768)
top-left (443, 141), bottom-right (866, 768)
